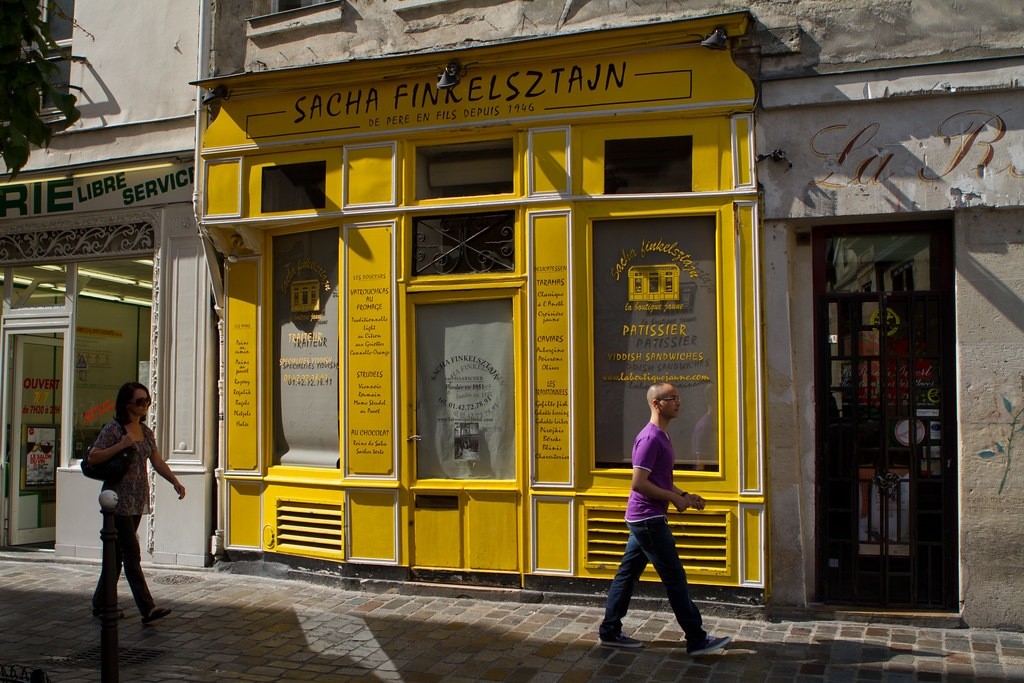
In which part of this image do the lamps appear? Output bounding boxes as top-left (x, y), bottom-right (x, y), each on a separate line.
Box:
top-left (701, 28), bottom-right (728, 50)
top-left (202, 86), bottom-right (228, 104)
top-left (437, 62), bottom-right (461, 89)
top-left (227, 234), bottom-right (244, 263)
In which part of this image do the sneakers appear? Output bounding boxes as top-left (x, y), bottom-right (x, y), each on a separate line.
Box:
top-left (687, 634), bottom-right (731, 656)
top-left (599, 631), bottom-right (643, 648)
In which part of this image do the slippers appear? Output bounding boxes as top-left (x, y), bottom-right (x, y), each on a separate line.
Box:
top-left (141, 607), bottom-right (171, 623)
top-left (92, 604), bottom-right (124, 618)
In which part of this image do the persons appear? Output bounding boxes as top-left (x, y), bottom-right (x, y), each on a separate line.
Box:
top-left (88, 382), bottom-right (185, 623)
top-left (691, 382), bottom-right (719, 471)
top-left (598, 383), bottom-right (732, 656)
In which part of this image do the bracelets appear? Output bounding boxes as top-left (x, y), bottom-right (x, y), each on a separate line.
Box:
top-left (681, 492), bottom-right (688, 496)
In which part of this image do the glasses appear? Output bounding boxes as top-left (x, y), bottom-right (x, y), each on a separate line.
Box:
top-left (128, 397), bottom-right (149, 406)
top-left (657, 395), bottom-right (680, 401)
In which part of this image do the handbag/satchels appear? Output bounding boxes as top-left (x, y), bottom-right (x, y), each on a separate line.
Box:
top-left (81, 418), bottom-right (136, 482)
